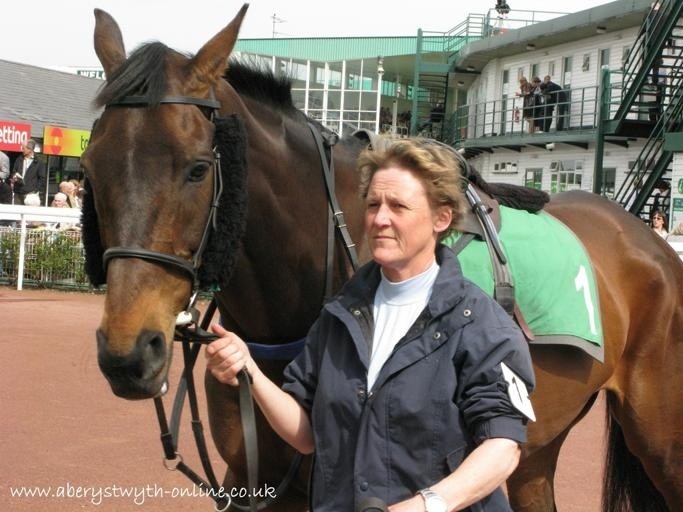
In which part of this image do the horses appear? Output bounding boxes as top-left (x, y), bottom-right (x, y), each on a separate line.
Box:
top-left (80, 2), bottom-right (683, 512)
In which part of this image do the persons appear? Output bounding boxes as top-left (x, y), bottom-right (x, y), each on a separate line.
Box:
top-left (1, 138), bottom-right (83, 209)
top-left (650, 179), bottom-right (669, 228)
top-left (203, 131), bottom-right (537, 512)
top-left (307, 93), bottom-right (442, 133)
top-left (523, 77), bottom-right (552, 132)
top-left (515, 77), bottom-right (531, 132)
top-left (540, 75), bottom-right (567, 132)
top-left (649, 211), bottom-right (668, 239)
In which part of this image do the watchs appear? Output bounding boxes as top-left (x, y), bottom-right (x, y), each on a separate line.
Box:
top-left (416, 487), bottom-right (446, 512)
